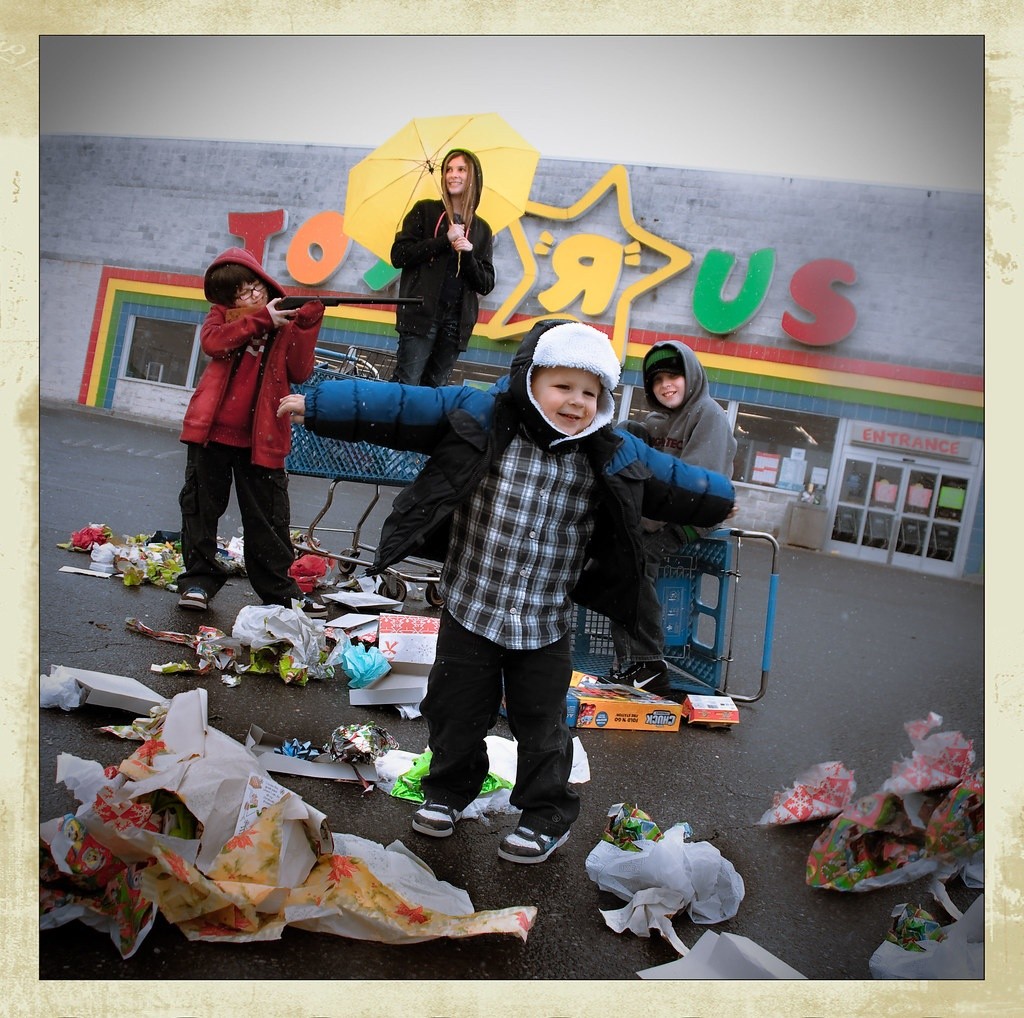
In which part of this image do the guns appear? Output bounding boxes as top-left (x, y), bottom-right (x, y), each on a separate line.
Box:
top-left (224, 295), bottom-right (426, 326)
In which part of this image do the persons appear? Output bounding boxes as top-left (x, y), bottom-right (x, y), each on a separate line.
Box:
top-left (175, 248), bottom-right (329, 619)
top-left (596, 337), bottom-right (738, 698)
top-left (389, 147), bottom-right (497, 387)
top-left (274, 318), bottom-right (741, 864)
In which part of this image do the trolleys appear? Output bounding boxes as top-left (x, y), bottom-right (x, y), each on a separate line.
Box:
top-left (283, 348), bottom-right (444, 609)
top-left (499, 524), bottom-right (787, 714)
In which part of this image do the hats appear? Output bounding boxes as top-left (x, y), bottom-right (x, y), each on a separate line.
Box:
top-left (510, 320), bottom-right (622, 455)
top-left (644, 345), bottom-right (686, 392)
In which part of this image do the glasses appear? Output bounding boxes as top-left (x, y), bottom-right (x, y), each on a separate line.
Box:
top-left (235, 282), bottom-right (265, 301)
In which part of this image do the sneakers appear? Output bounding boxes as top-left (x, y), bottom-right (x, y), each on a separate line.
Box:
top-left (497, 827), bottom-right (571, 864)
top-left (412, 798), bottom-right (463, 837)
top-left (178, 585), bottom-right (208, 610)
top-left (272, 593), bottom-right (328, 618)
top-left (609, 662), bottom-right (670, 693)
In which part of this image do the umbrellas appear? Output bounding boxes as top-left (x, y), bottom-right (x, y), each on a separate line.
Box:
top-left (340, 111), bottom-right (540, 268)
